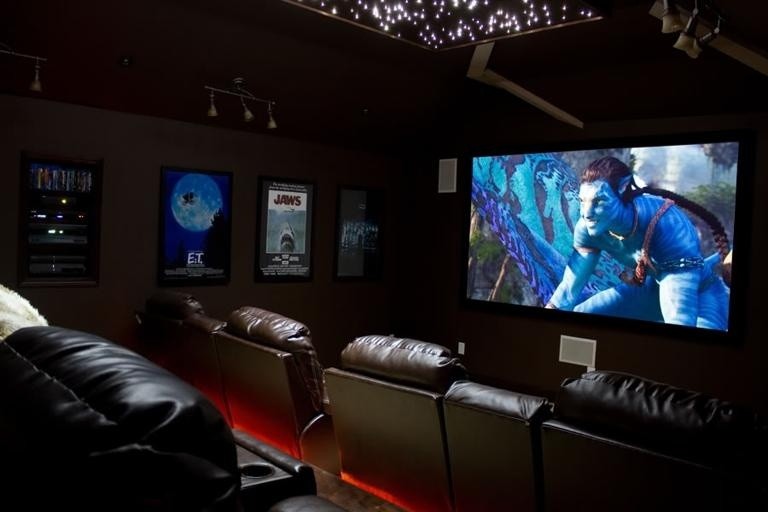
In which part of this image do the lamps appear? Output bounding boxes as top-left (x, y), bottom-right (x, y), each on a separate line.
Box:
top-left (205, 78), bottom-right (279, 130)
top-left (659, 1), bottom-right (724, 59)
top-left (29, 57), bottom-right (43, 91)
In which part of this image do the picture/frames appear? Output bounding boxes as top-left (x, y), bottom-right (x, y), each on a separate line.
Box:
top-left (255, 175), bottom-right (317, 284)
top-left (156, 165), bottom-right (233, 287)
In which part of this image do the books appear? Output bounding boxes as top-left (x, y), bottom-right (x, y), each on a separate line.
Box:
top-left (35, 166), bottom-right (92, 192)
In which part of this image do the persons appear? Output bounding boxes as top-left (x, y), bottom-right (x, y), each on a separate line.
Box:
top-left (545, 156), bottom-right (727, 331)
